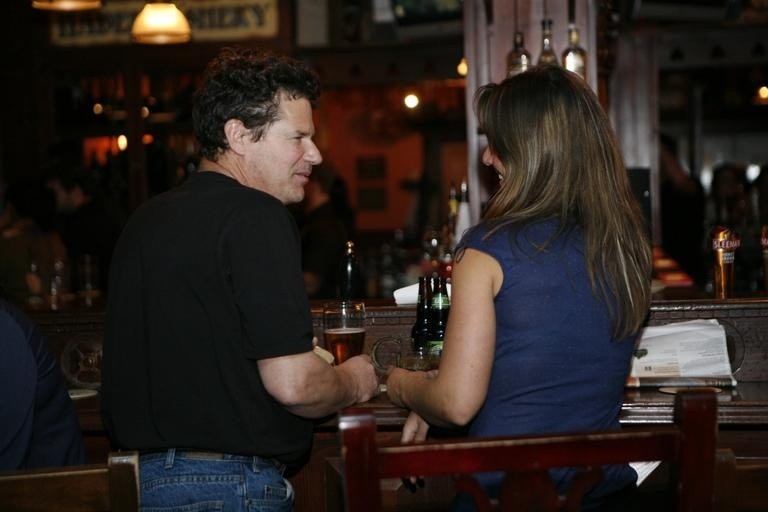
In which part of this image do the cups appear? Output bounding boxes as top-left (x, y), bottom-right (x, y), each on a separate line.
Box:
top-left (323, 301), bottom-right (366, 364)
top-left (400, 337), bottom-right (431, 372)
top-left (711, 226), bottom-right (735, 299)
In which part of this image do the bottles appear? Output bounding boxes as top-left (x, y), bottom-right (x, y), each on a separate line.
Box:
top-left (539, 20), bottom-right (558, 68)
top-left (81, 71), bottom-right (156, 178)
top-left (507, 32), bottom-right (532, 78)
top-left (416, 180), bottom-right (473, 359)
top-left (563, 26), bottom-right (587, 80)
top-left (342, 240), bottom-right (359, 301)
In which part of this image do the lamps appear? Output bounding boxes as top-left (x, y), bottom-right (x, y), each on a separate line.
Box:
top-left (130, 3), bottom-right (192, 46)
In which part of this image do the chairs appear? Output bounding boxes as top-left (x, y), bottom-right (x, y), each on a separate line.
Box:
top-left (1, 451), bottom-right (140, 509)
top-left (336, 388), bottom-right (736, 512)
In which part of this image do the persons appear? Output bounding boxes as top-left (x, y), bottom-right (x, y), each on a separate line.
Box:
top-left (658, 135), bottom-right (768, 276)
top-left (292, 164), bottom-right (356, 301)
top-left (387, 63), bottom-right (653, 512)
top-left (1, 297), bottom-right (87, 470)
top-left (0, 170), bottom-right (109, 310)
top-left (95, 47), bottom-right (380, 512)
